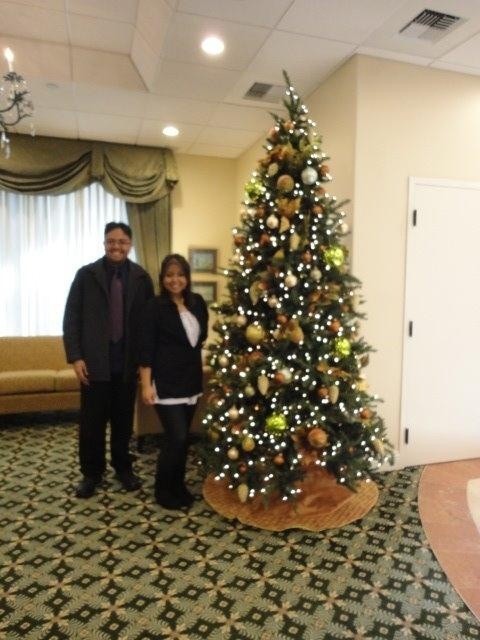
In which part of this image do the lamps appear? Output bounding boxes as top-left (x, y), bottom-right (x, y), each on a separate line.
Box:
top-left (0, 46), bottom-right (36, 159)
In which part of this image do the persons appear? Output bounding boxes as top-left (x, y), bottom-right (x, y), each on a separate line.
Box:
top-left (62, 223), bottom-right (156, 496)
top-left (137, 251), bottom-right (208, 510)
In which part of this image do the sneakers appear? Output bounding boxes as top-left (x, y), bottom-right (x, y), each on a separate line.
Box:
top-left (76, 473), bottom-right (102, 498)
top-left (171, 487), bottom-right (195, 506)
top-left (115, 469), bottom-right (140, 491)
top-left (157, 493), bottom-right (184, 509)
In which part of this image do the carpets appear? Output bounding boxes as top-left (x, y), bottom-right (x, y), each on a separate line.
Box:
top-left (202, 469), bottom-right (378, 533)
top-left (418, 457), bottom-right (479, 619)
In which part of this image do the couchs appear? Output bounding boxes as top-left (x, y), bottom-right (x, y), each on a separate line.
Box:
top-left (0, 336), bottom-right (82, 416)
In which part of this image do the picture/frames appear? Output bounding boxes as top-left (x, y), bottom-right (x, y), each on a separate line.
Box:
top-left (188, 248), bottom-right (218, 305)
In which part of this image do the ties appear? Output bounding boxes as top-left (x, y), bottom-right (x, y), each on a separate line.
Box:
top-left (108, 269), bottom-right (124, 344)
